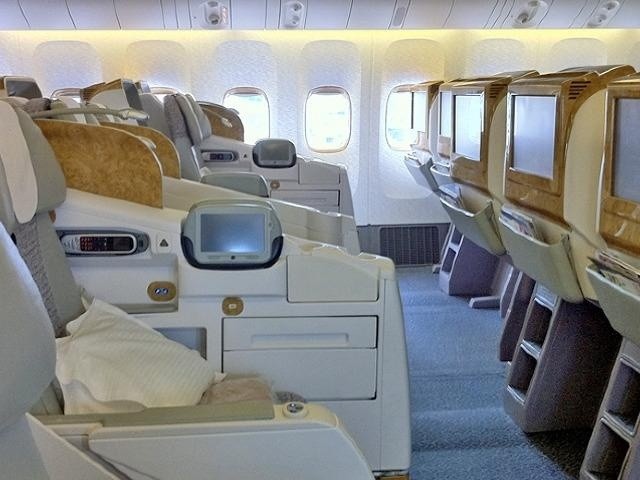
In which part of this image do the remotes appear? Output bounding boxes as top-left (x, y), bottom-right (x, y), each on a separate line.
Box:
top-left (60, 233), bottom-right (138, 255)
top-left (202, 151), bottom-right (235, 161)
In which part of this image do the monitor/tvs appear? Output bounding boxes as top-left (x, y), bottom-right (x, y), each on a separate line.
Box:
top-left (200, 172), bottom-right (269, 197)
top-left (453, 94), bottom-right (481, 162)
top-left (253, 139), bottom-right (296, 168)
top-left (411, 91), bottom-right (426, 133)
top-left (439, 91), bottom-right (451, 138)
top-left (182, 197), bottom-right (283, 269)
top-left (509, 94), bottom-right (556, 181)
top-left (610, 97), bottom-right (640, 204)
